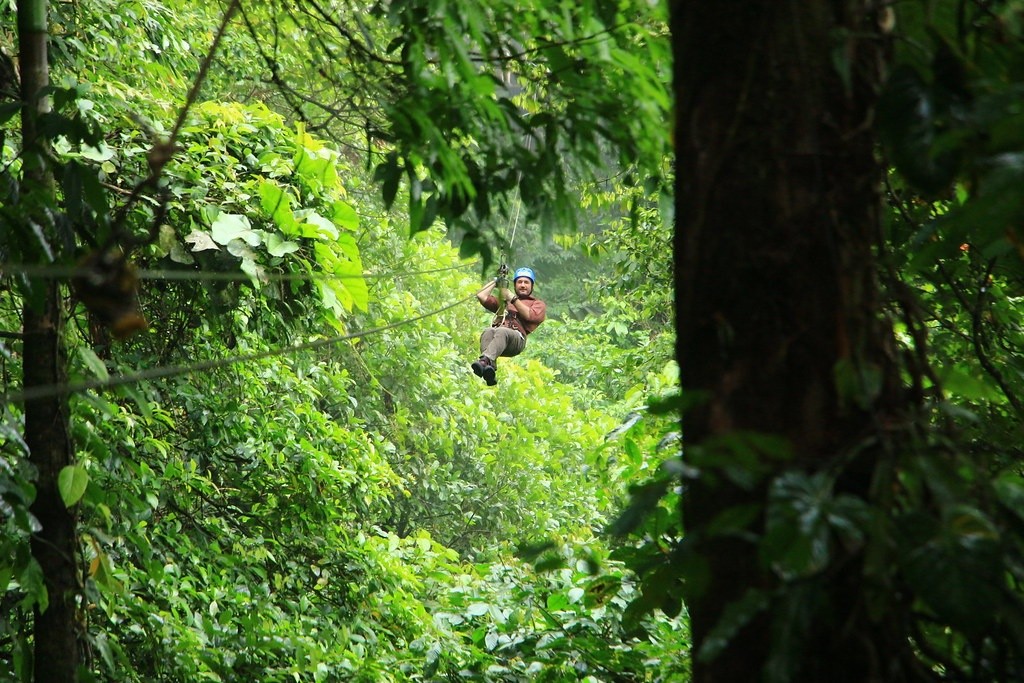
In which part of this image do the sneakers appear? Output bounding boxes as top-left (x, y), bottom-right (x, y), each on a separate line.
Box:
top-left (472, 361), bottom-right (484, 377)
top-left (483, 365), bottom-right (497, 387)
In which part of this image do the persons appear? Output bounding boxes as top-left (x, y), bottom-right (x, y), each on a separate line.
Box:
top-left (472, 267), bottom-right (547, 388)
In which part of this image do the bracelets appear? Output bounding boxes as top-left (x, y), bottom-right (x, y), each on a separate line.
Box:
top-left (510, 295), bottom-right (517, 304)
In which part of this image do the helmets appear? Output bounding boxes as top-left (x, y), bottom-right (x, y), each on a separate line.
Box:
top-left (513, 267), bottom-right (535, 283)
